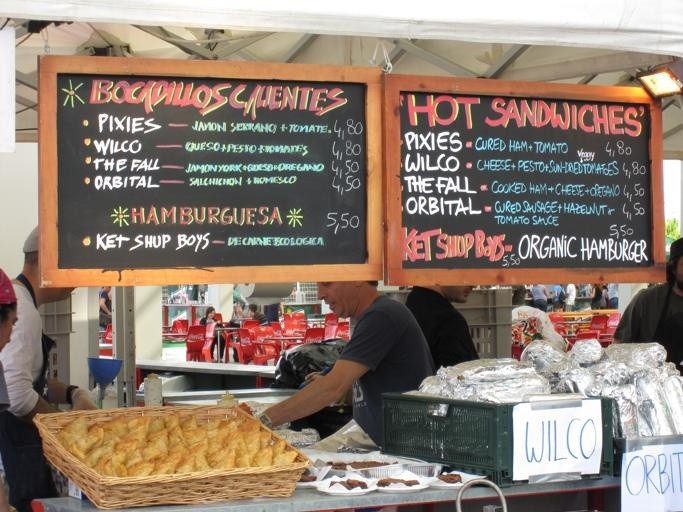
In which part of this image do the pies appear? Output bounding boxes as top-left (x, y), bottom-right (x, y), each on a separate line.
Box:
top-left (49, 412), bottom-right (299, 479)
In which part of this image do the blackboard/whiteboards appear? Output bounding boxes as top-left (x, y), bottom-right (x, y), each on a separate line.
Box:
top-left (381, 73), bottom-right (668, 287)
top-left (37, 54), bottom-right (384, 289)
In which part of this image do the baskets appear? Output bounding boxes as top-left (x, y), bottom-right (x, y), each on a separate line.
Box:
top-left (380, 390), bottom-right (613, 488)
top-left (31, 404), bottom-right (313, 511)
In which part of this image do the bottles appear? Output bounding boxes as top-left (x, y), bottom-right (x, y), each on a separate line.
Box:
top-left (216, 391), bottom-right (238, 407)
top-left (142, 373), bottom-right (163, 407)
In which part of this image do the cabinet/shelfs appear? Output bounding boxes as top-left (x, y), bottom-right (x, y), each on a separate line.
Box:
top-left (37, 293), bottom-right (76, 386)
top-left (134, 363), bottom-right (273, 408)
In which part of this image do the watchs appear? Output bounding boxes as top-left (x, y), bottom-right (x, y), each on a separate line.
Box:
top-left (257, 412), bottom-right (276, 430)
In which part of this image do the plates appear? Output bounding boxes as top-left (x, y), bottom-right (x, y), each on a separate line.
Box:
top-left (312, 477), bottom-right (378, 497)
top-left (372, 479), bottom-right (430, 494)
top-left (428, 479), bottom-right (465, 491)
top-left (295, 481), bottom-right (324, 489)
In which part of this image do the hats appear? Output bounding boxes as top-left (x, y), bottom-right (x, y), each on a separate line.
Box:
top-left (0, 268), bottom-right (17, 305)
top-left (669, 237), bottom-right (682, 259)
top-left (23, 224), bottom-right (39, 253)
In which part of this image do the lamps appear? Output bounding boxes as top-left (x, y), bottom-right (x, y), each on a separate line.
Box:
top-left (633, 57), bottom-right (683, 101)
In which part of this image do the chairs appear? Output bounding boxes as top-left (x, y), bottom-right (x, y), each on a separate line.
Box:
top-left (170, 309), bottom-right (349, 365)
top-left (549, 308), bottom-right (622, 348)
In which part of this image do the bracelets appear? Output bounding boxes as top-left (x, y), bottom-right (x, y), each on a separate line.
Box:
top-left (64, 384), bottom-right (79, 408)
top-left (69, 385), bottom-right (94, 407)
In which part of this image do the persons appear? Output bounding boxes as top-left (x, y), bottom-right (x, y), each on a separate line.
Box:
top-left (198, 300), bottom-right (281, 364)
top-left (611, 237), bottom-right (683, 375)
top-left (99, 286), bottom-right (112, 333)
top-left (0, 267), bottom-right (18, 512)
top-left (253, 280), bottom-right (436, 449)
top-left (0, 224), bottom-right (98, 512)
top-left (405, 285), bottom-right (480, 372)
top-left (511, 284), bottom-right (619, 311)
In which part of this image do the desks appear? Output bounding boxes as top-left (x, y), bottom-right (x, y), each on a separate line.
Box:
top-left (19, 468), bottom-right (622, 512)
